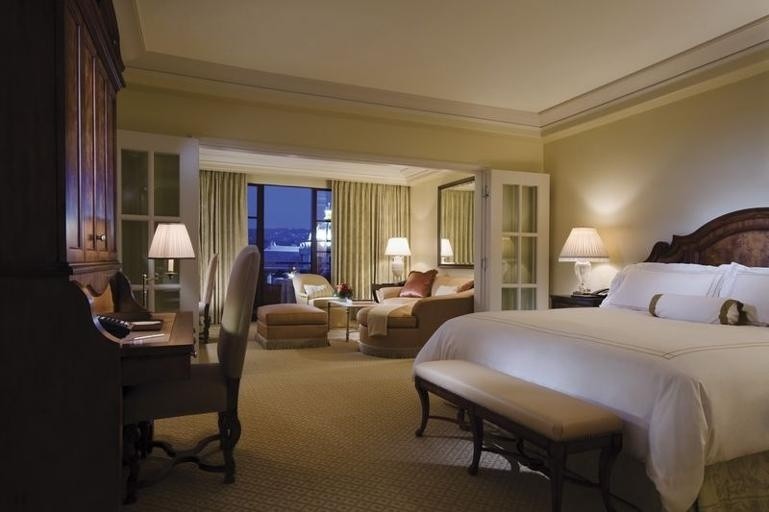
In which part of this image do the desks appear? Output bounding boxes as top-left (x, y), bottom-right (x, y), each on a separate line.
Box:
top-left (370, 281), bottom-right (408, 303)
top-left (121, 310), bottom-right (196, 385)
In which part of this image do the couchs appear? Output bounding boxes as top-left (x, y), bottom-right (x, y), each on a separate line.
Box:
top-left (256, 302), bottom-right (330, 350)
top-left (293, 274), bottom-right (351, 330)
top-left (359, 270), bottom-right (474, 358)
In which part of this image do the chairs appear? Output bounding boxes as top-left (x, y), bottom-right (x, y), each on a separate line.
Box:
top-left (120, 243), bottom-right (262, 505)
top-left (198, 250), bottom-right (219, 343)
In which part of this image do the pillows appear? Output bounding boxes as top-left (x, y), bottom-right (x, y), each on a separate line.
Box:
top-left (600, 262), bottom-right (718, 322)
top-left (401, 270), bottom-right (438, 297)
top-left (647, 292), bottom-right (746, 325)
top-left (432, 272), bottom-right (474, 296)
top-left (710, 260), bottom-right (768, 325)
top-left (304, 284), bottom-right (333, 298)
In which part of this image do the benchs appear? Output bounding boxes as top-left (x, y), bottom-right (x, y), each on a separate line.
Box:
top-left (413, 358), bottom-right (627, 511)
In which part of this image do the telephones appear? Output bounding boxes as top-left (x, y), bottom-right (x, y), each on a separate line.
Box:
top-left (592, 288), bottom-right (609, 296)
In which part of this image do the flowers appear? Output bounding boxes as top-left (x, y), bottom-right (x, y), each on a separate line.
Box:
top-left (336, 282), bottom-right (353, 298)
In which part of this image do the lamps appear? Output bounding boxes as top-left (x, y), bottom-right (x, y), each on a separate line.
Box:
top-left (146, 222), bottom-right (195, 281)
top-left (558, 227), bottom-right (610, 295)
top-left (384, 236), bottom-right (411, 283)
top-left (440, 238), bottom-right (455, 264)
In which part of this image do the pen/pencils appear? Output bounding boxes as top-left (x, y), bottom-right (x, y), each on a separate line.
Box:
top-left (133, 333), bottom-right (165, 340)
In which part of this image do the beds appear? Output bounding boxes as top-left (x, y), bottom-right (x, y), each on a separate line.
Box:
top-left (417, 206), bottom-right (768, 512)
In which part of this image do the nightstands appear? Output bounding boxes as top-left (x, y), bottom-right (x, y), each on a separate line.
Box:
top-left (549, 293), bottom-right (605, 308)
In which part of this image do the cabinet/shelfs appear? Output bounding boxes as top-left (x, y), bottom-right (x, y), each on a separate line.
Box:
top-left (0, 4), bottom-right (196, 510)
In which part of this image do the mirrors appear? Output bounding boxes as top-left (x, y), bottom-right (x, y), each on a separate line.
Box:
top-left (437, 175), bottom-right (476, 268)
